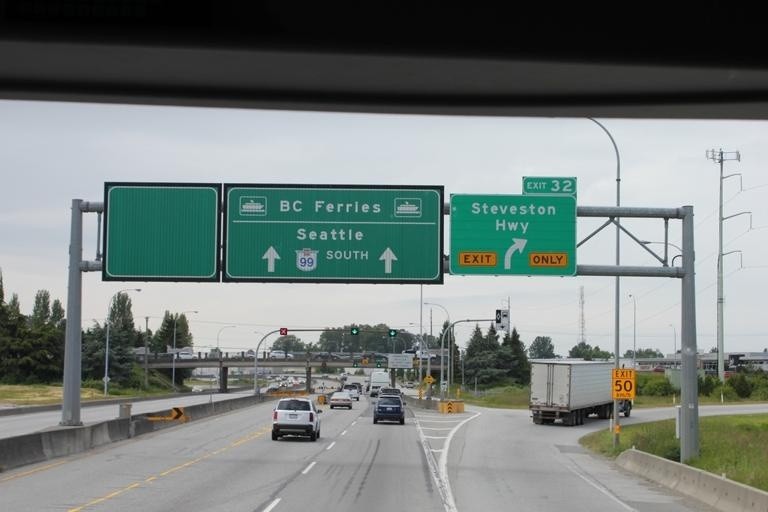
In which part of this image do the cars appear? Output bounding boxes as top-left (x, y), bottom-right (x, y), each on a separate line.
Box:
top-left (271, 350), bottom-right (293, 357)
top-left (402, 350), bottom-right (437, 361)
top-left (329, 382), bottom-right (406, 424)
top-left (407, 382), bottom-right (413, 388)
top-left (267, 376), bottom-right (305, 392)
top-left (174, 352), bottom-right (198, 359)
top-left (314, 352), bottom-right (340, 360)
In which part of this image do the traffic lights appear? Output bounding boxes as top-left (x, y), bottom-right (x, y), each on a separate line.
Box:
top-left (389, 330), bottom-right (396, 337)
top-left (279, 328), bottom-right (287, 335)
top-left (350, 327), bottom-right (359, 336)
top-left (353, 360), bottom-right (358, 368)
top-left (377, 360), bottom-right (381, 368)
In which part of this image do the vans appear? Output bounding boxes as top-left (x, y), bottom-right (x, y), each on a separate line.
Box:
top-left (272, 397), bottom-right (323, 441)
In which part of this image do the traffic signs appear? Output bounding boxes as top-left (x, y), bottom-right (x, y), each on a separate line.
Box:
top-left (449, 194), bottom-right (578, 277)
top-left (225, 183), bottom-right (444, 281)
top-left (612, 369), bottom-right (636, 400)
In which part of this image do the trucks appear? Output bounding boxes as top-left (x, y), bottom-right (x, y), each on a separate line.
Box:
top-left (528, 358), bottom-right (633, 425)
top-left (370, 372), bottom-right (391, 397)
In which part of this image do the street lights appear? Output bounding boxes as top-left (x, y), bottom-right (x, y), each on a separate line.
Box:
top-left (670, 324), bottom-right (677, 368)
top-left (172, 310), bottom-right (198, 384)
top-left (629, 294), bottom-right (635, 358)
top-left (105, 288), bottom-right (142, 395)
top-left (642, 241), bottom-right (682, 252)
top-left (216, 325), bottom-right (235, 351)
top-left (423, 302), bottom-right (453, 383)
